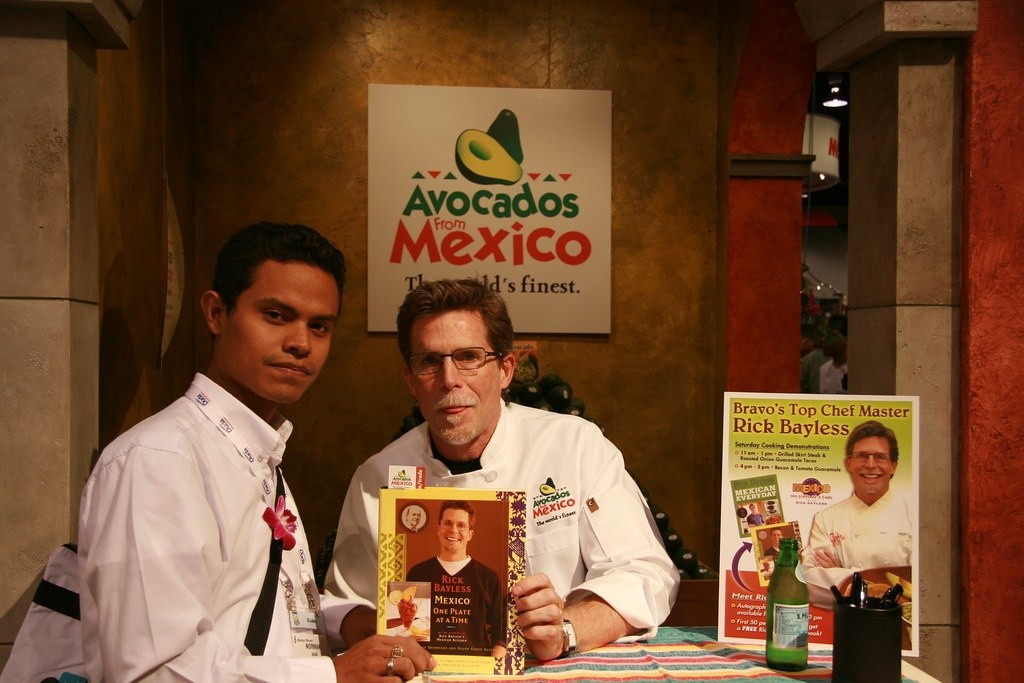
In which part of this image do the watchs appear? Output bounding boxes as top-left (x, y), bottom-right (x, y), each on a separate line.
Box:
top-left (558, 618), bottom-right (576, 659)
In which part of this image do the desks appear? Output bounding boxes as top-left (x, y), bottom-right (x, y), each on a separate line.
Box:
top-left (338, 625), bottom-right (941, 682)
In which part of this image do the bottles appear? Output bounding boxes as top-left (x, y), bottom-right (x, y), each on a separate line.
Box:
top-left (766, 538), bottom-right (809, 671)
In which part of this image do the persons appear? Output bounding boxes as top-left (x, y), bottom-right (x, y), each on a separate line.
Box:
top-left (77, 223), bottom-right (436, 683)
top-left (324, 279), bottom-right (680, 663)
top-left (800, 313), bottom-right (847, 395)
top-left (407, 500), bottom-right (507, 657)
top-left (746, 503), bottom-right (765, 529)
top-left (799, 419), bottom-right (913, 612)
top-left (764, 527), bottom-right (784, 559)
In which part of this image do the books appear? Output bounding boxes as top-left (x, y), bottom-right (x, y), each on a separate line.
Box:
top-left (376, 485), bottom-right (527, 677)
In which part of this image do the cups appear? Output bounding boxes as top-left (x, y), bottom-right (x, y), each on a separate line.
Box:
top-left (832, 596), bottom-right (903, 683)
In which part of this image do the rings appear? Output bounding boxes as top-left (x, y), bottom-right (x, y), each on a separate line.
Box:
top-left (387, 658), bottom-right (394, 674)
top-left (391, 644), bottom-right (403, 657)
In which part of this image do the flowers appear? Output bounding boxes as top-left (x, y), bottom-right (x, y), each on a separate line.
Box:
top-left (262, 494), bottom-right (298, 550)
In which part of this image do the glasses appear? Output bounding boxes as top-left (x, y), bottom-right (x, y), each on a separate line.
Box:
top-left (406, 347), bottom-right (505, 374)
top-left (847, 451), bottom-right (892, 463)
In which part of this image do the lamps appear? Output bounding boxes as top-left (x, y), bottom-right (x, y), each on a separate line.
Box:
top-left (819, 74), bottom-right (849, 106)
